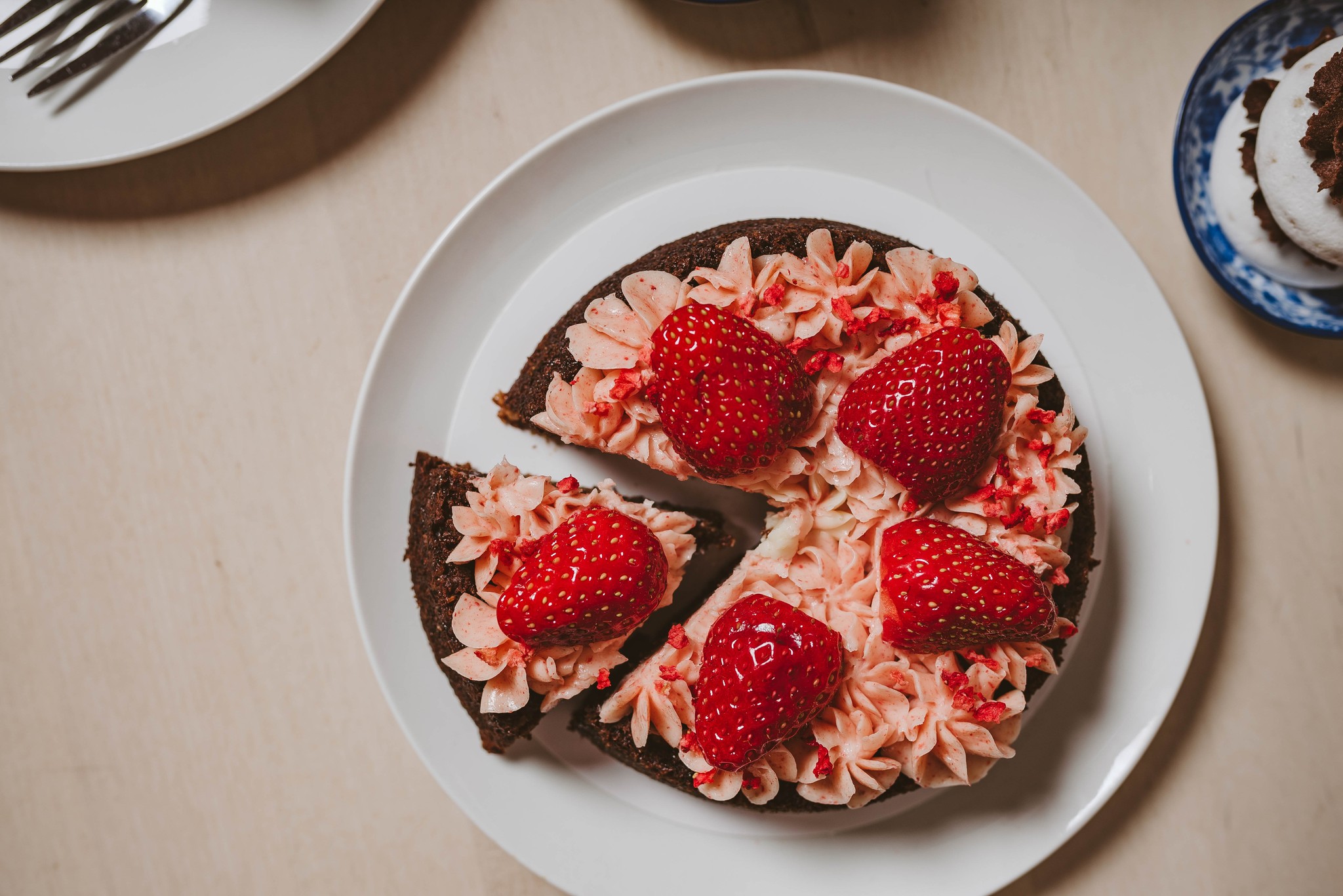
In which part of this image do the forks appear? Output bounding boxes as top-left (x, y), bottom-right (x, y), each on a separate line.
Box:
top-left (0, 0), bottom-right (193, 98)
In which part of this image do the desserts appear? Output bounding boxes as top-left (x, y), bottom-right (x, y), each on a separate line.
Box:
top-left (1238, 26), bottom-right (1343, 273)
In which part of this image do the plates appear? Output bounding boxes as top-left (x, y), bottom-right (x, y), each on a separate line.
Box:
top-left (0, 0), bottom-right (382, 173)
top-left (1170, 0), bottom-right (1342, 340)
top-left (342, 67), bottom-right (1224, 896)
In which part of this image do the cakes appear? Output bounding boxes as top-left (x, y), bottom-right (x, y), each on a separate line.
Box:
top-left (494, 214), bottom-right (1095, 812)
top-left (408, 450), bottom-right (725, 756)
